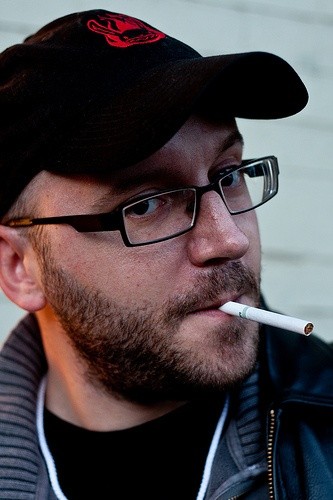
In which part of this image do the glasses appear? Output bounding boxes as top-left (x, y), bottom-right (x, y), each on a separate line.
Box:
top-left (0, 155), bottom-right (280, 248)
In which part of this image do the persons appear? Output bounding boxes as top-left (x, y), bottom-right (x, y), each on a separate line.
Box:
top-left (0, 9), bottom-right (333, 500)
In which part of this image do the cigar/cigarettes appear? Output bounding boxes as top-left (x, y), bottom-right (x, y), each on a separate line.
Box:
top-left (219, 301), bottom-right (316, 337)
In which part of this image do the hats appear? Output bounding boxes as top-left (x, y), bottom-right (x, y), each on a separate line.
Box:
top-left (0, 9), bottom-right (309, 215)
